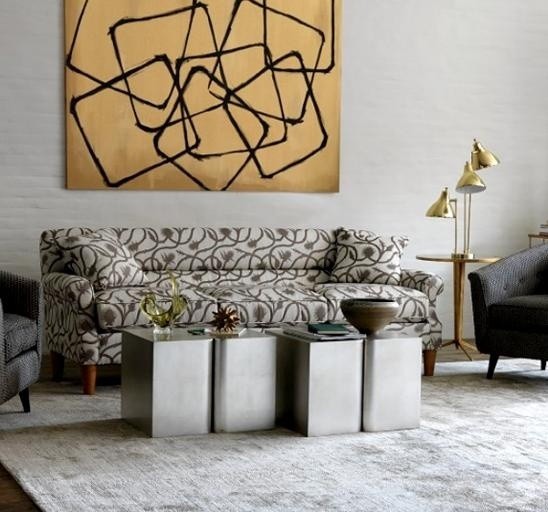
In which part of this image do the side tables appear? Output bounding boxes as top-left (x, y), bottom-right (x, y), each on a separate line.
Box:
top-left (416, 255), bottom-right (501, 362)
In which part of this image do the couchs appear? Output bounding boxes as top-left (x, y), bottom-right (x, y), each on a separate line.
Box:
top-left (40, 226), bottom-right (445, 396)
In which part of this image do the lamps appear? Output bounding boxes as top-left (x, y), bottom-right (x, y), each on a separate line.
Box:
top-left (425, 138), bottom-right (500, 258)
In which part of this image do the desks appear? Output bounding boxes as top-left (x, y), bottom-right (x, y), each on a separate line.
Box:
top-left (527, 234), bottom-right (548, 246)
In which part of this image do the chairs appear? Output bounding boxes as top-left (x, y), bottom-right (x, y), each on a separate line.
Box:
top-left (0, 271), bottom-right (41, 414)
top-left (467, 243), bottom-right (548, 380)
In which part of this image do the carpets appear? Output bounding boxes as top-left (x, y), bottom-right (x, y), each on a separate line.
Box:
top-left (1, 357), bottom-right (548, 511)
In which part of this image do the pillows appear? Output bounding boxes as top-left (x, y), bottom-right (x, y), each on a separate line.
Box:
top-left (53, 227), bottom-right (149, 291)
top-left (329, 227), bottom-right (410, 284)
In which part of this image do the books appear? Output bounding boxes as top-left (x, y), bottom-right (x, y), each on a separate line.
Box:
top-left (210, 327), bottom-right (247, 336)
top-left (283, 327), bottom-right (366, 341)
top-left (308, 324), bottom-right (349, 335)
